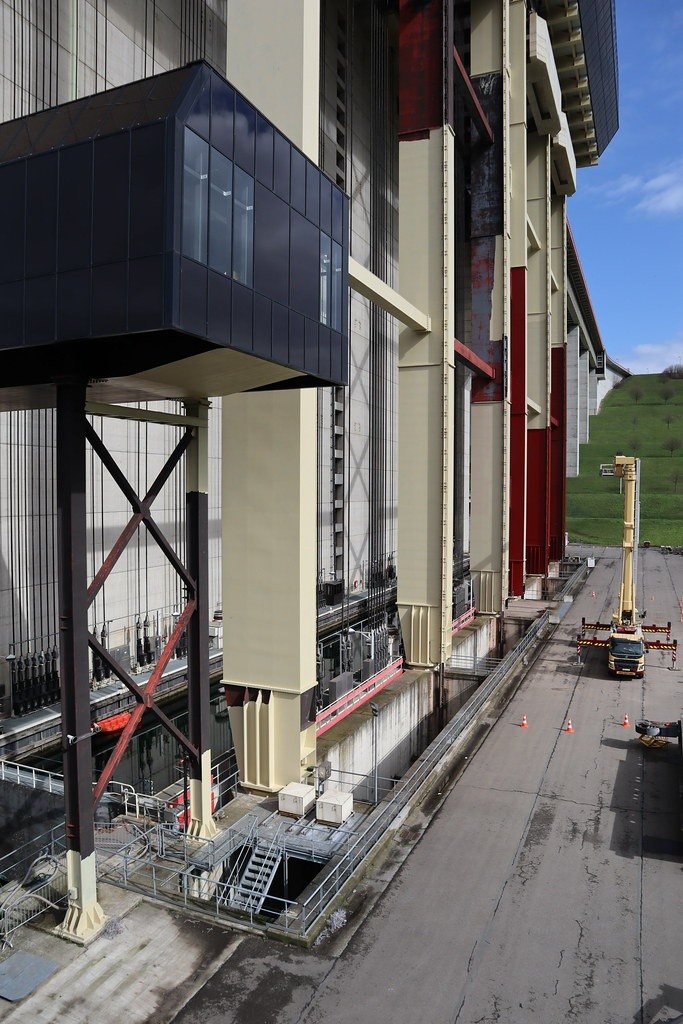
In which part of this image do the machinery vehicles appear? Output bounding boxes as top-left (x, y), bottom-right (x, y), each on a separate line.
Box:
top-left (598, 452), bottom-right (649, 681)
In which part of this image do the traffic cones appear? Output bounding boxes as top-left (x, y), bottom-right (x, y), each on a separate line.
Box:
top-left (519, 713), bottom-right (529, 728)
top-left (622, 712), bottom-right (632, 727)
top-left (564, 718), bottom-right (575, 733)
